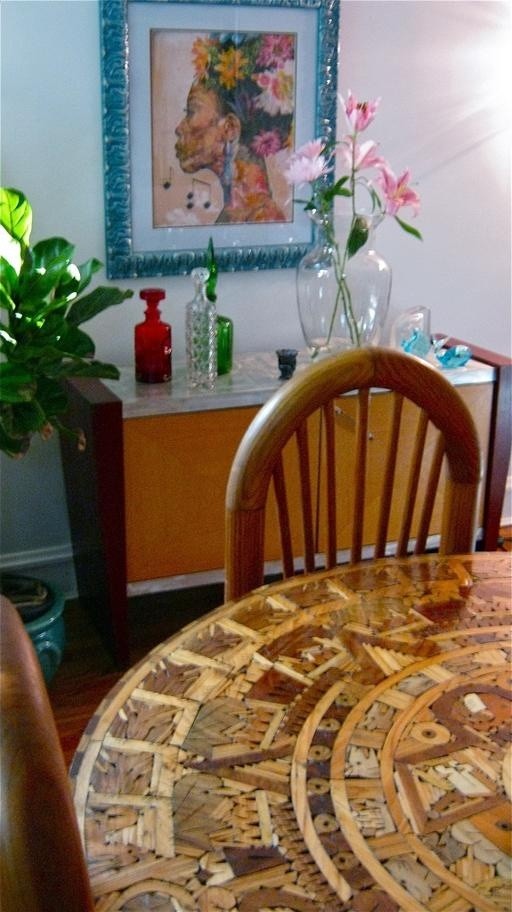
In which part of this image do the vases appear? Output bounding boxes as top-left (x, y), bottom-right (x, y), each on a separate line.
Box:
top-left (295, 209), bottom-right (392, 358)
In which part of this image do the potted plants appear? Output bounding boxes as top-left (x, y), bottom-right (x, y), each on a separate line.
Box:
top-left (0, 186), bottom-right (133, 691)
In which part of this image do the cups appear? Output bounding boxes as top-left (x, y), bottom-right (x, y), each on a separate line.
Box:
top-left (276, 348), bottom-right (298, 380)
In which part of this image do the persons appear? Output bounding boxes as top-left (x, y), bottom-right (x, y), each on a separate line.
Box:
top-left (173, 32), bottom-right (296, 226)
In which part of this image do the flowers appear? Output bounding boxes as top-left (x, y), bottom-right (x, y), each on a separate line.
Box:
top-left (283, 88), bottom-right (424, 347)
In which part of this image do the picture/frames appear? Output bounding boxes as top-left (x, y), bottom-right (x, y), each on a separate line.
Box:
top-left (98, 1), bottom-right (340, 281)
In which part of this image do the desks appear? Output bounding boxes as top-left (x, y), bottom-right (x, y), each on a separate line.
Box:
top-left (57, 333), bottom-right (512, 651)
top-left (69, 551), bottom-right (512, 912)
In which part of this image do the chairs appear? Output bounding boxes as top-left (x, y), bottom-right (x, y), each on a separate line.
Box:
top-left (223, 349), bottom-right (479, 602)
top-left (1, 596), bottom-right (93, 912)
